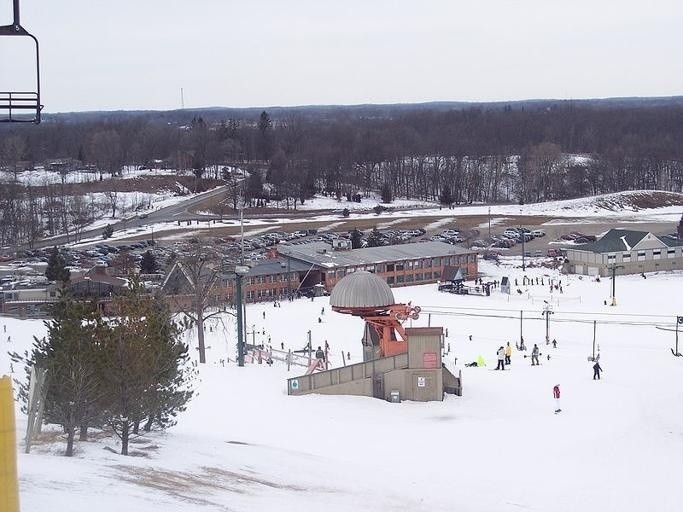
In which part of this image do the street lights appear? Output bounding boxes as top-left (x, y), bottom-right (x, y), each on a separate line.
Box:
top-left (237, 187), bottom-right (244, 265)
top-left (487, 205), bottom-right (491, 234)
top-left (515, 226), bottom-right (525, 272)
top-left (518, 207), bottom-right (523, 229)
top-left (232, 263), bottom-right (253, 369)
top-left (602, 262), bottom-right (625, 307)
top-left (538, 299), bottom-right (556, 346)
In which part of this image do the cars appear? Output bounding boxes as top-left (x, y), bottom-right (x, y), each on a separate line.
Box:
top-left (520, 247), bottom-right (567, 260)
top-left (554, 230), bottom-right (597, 245)
top-left (469, 226), bottom-right (546, 249)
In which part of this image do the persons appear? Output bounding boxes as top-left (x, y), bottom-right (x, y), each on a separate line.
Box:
top-left (7, 336), bottom-right (10, 343)
top-left (593, 362), bottom-right (602, 380)
top-left (180, 286), bottom-right (329, 370)
top-left (475, 274), bottom-right (564, 294)
top-left (551, 338), bottom-right (557, 348)
top-left (494, 347), bottom-right (505, 370)
top-left (531, 344), bottom-right (539, 366)
top-left (4, 325), bottom-right (7, 333)
top-left (553, 383), bottom-right (561, 413)
top-left (505, 341), bottom-right (512, 364)
